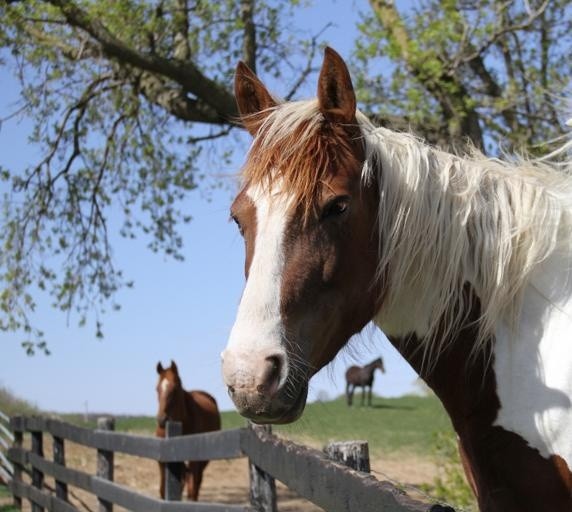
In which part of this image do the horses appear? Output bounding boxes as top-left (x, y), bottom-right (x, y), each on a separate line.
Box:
top-left (345, 358), bottom-right (385, 406)
top-left (218, 45), bottom-right (572, 512)
top-left (155, 360), bottom-right (221, 502)
